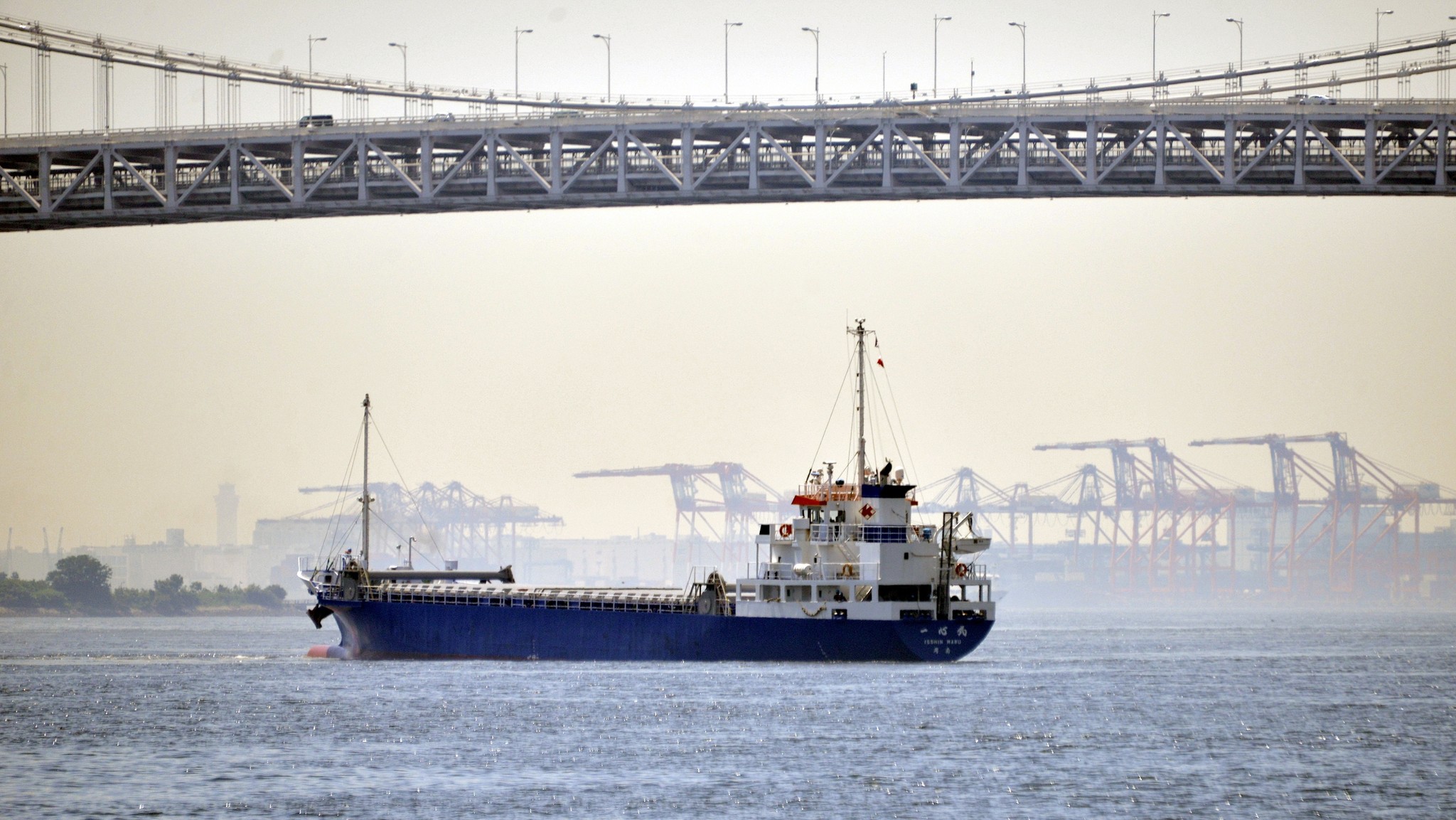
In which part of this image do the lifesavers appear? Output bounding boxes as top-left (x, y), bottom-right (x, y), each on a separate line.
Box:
top-left (841, 564), bottom-right (852, 576)
top-left (955, 563), bottom-right (967, 576)
top-left (779, 524), bottom-right (791, 538)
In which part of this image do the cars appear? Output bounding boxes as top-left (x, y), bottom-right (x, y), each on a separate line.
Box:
top-left (1286, 94), bottom-right (1337, 105)
top-left (427, 112), bottom-right (456, 123)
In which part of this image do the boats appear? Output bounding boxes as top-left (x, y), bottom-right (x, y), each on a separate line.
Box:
top-left (291, 316), bottom-right (998, 665)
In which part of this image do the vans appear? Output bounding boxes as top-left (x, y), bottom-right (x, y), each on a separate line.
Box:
top-left (299, 115), bottom-right (334, 127)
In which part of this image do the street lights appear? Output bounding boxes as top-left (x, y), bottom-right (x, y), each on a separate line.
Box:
top-left (187, 51), bottom-right (206, 130)
top-left (1153, 10), bottom-right (1171, 101)
top-left (1008, 21), bottom-right (1027, 104)
top-left (1226, 16), bottom-right (1244, 104)
top-left (514, 26), bottom-right (533, 115)
top-left (1376, 7), bottom-right (1395, 98)
top-left (387, 41), bottom-right (408, 123)
top-left (802, 26), bottom-right (820, 103)
top-left (593, 33), bottom-right (612, 117)
top-left (933, 13), bottom-right (952, 98)
top-left (307, 34), bottom-right (328, 127)
top-left (724, 19), bottom-right (743, 105)
